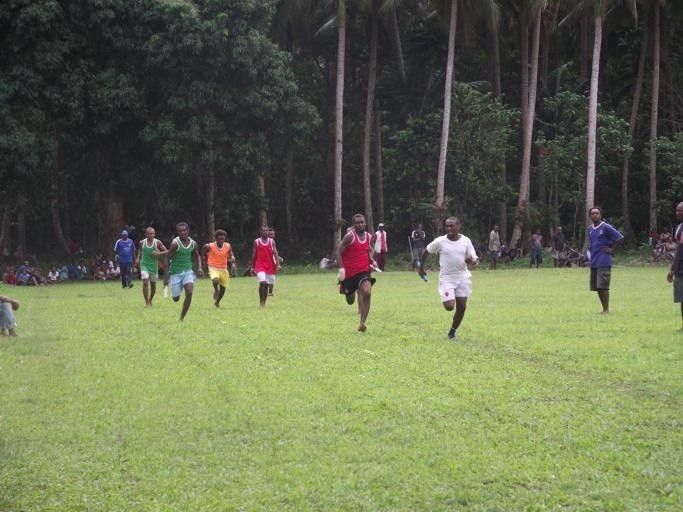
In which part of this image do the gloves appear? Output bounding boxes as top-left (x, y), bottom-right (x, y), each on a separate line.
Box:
top-left (373, 261), bottom-right (378, 270)
top-left (337, 267), bottom-right (345, 282)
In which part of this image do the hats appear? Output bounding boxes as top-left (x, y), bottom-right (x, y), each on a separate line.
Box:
top-left (122, 230), bottom-right (128, 236)
top-left (378, 223), bottom-right (385, 229)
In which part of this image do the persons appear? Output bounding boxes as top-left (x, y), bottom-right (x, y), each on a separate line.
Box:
top-left (0, 295), bottom-right (22, 337)
top-left (418, 217), bottom-right (480, 340)
top-left (647, 226), bottom-right (677, 264)
top-left (588, 208), bottom-right (624, 315)
top-left (318, 252), bottom-right (337, 270)
top-left (528, 227), bottom-right (542, 268)
top-left (226, 248), bottom-right (257, 278)
top-left (108, 256), bottom-right (121, 284)
top-left (131, 252), bottom-right (162, 279)
top-left (202, 229), bottom-right (236, 310)
top-left (488, 224), bottom-right (500, 270)
top-left (472, 239), bottom-right (509, 262)
top-left (0, 240), bottom-right (107, 287)
top-left (249, 227), bottom-right (284, 306)
top-left (136, 227), bottom-right (169, 306)
top-left (164, 222), bottom-right (201, 322)
top-left (557, 245), bottom-right (591, 268)
top-left (335, 214), bottom-right (388, 333)
top-left (552, 226), bottom-right (566, 267)
top-left (125, 219), bottom-right (159, 239)
top-left (515, 239), bottom-right (525, 259)
top-left (667, 201), bottom-right (683, 335)
top-left (113, 230), bottom-right (137, 289)
top-left (200, 249), bottom-right (207, 278)
top-left (373, 223), bottom-right (387, 271)
top-left (410, 224), bottom-right (427, 273)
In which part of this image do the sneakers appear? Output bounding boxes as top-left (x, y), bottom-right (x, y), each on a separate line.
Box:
top-left (340, 284), bottom-right (346, 294)
top-left (124, 287), bottom-right (129, 288)
top-left (129, 283), bottom-right (133, 288)
top-left (9, 328), bottom-right (18, 336)
top-left (359, 324), bottom-right (366, 331)
top-left (1, 328), bottom-right (9, 336)
top-left (448, 327), bottom-right (457, 342)
